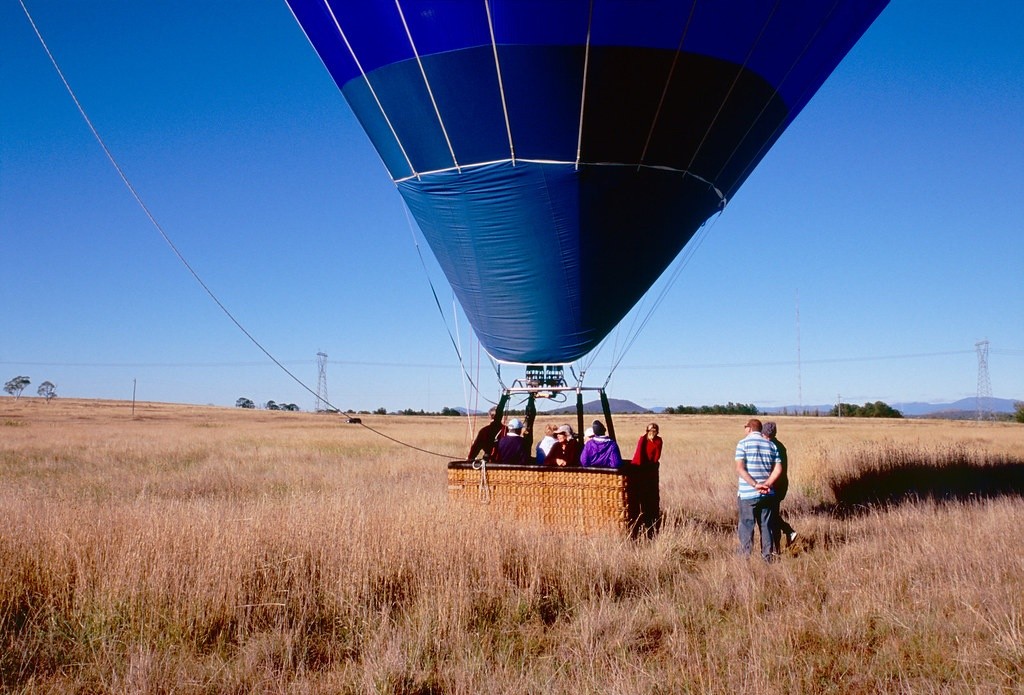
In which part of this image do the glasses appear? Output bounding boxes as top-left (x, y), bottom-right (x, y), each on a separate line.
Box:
top-left (745, 425), bottom-right (750, 427)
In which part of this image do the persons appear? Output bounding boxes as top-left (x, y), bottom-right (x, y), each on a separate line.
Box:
top-left (761, 423), bottom-right (797, 557)
top-left (735, 419), bottom-right (782, 564)
top-left (467, 406), bottom-right (622, 470)
top-left (630, 422), bottom-right (663, 467)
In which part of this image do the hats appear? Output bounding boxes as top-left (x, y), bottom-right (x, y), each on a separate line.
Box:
top-left (585, 427), bottom-right (595, 436)
top-left (551, 424), bottom-right (571, 441)
top-left (508, 418), bottom-right (525, 429)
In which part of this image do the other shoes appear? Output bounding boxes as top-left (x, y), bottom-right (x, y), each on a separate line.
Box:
top-left (785, 531), bottom-right (798, 547)
